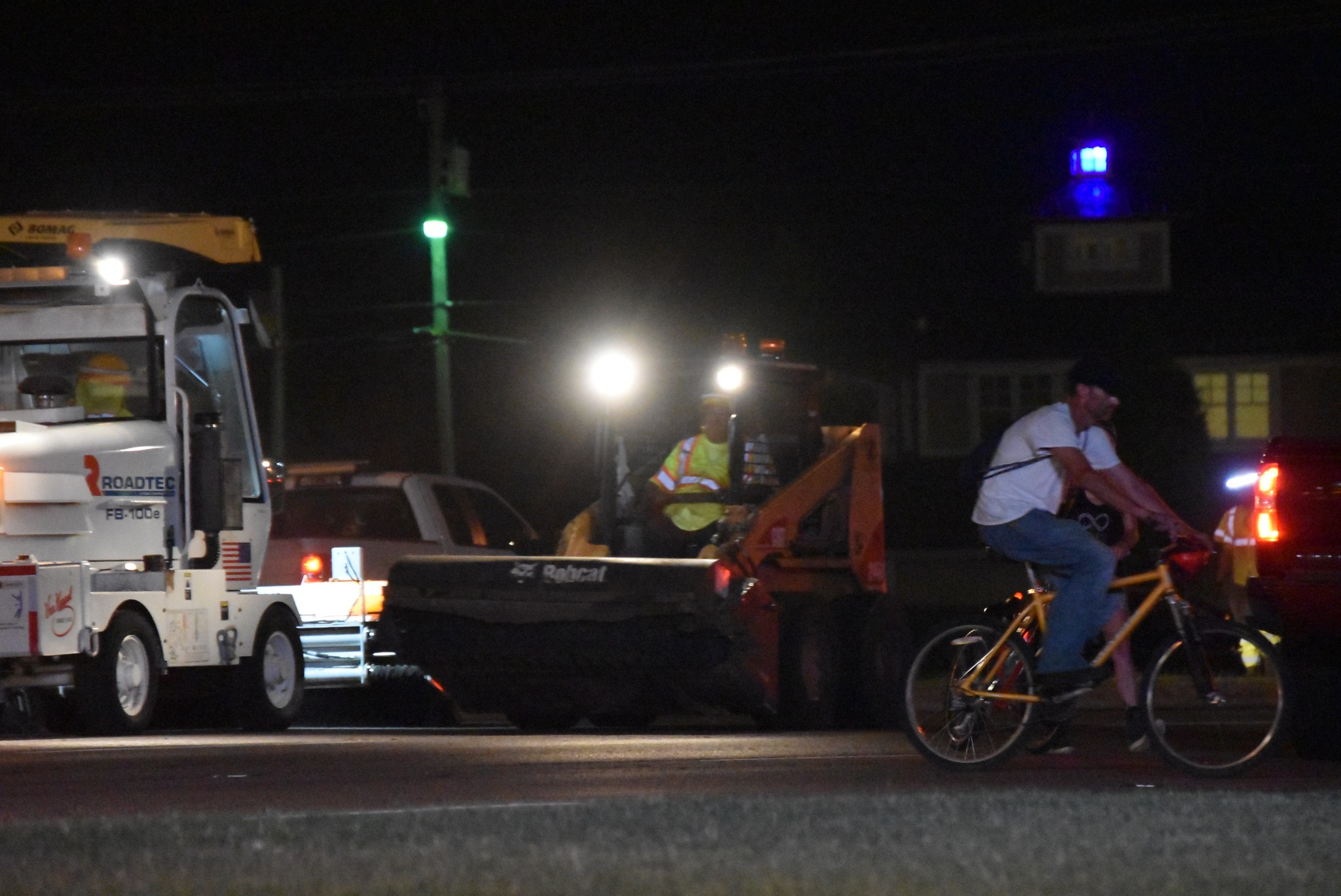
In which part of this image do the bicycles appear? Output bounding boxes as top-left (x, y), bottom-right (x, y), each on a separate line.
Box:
top-left (901, 540), bottom-right (1289, 773)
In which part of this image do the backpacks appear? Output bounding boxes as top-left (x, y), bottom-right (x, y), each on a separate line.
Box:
top-left (953, 429), bottom-right (1088, 499)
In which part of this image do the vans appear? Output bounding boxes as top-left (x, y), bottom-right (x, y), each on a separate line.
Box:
top-left (1251, 354), bottom-right (1341, 760)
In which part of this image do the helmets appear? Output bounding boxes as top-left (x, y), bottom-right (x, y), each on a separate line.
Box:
top-left (79, 353), bottom-right (134, 384)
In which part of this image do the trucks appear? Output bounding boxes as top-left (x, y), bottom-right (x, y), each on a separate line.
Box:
top-left (0, 210), bottom-right (452, 734)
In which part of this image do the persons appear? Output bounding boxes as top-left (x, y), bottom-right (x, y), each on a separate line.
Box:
top-left (649, 410), bottom-right (777, 561)
top-left (968, 361), bottom-right (1257, 757)
top-left (76, 350), bottom-right (135, 417)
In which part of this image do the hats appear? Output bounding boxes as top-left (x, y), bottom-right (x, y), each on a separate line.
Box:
top-left (1068, 353), bottom-right (1129, 397)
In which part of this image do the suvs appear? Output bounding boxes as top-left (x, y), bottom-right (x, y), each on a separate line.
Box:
top-left (263, 469), bottom-right (547, 585)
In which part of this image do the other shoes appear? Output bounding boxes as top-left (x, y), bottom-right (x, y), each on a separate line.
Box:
top-left (1051, 672), bottom-right (1093, 704)
top-left (1041, 740), bottom-right (1073, 755)
top-left (1126, 719), bottom-right (1166, 753)
